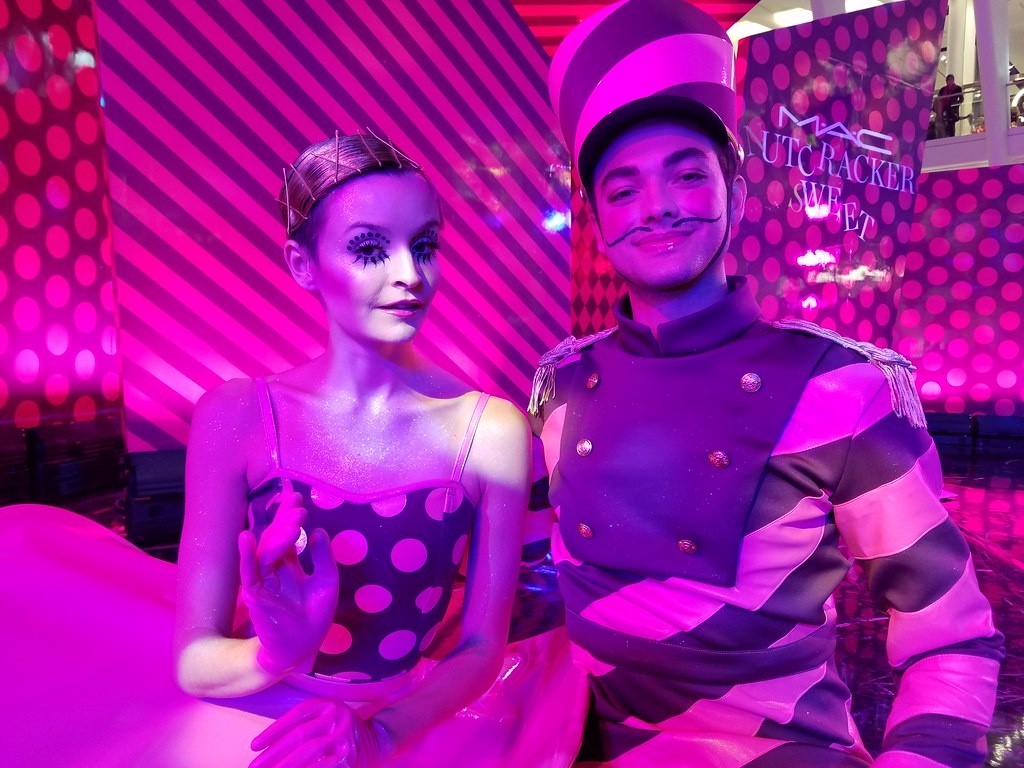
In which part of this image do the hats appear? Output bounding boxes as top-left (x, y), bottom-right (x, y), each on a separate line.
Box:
top-left (546, 0), bottom-right (745, 203)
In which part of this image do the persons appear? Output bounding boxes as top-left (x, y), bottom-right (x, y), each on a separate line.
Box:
top-left (532, 1), bottom-right (1003, 768)
top-left (937, 74), bottom-right (964, 137)
top-left (0, 133), bottom-right (590, 768)
